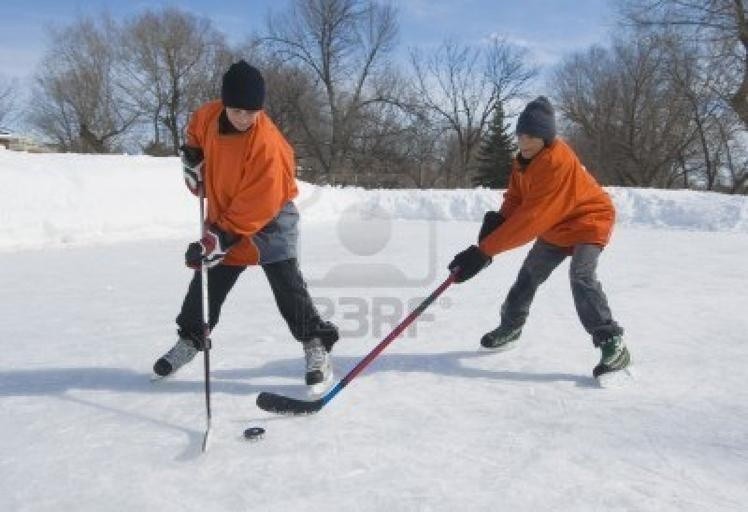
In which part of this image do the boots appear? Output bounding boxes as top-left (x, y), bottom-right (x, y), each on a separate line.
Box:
top-left (592, 335), bottom-right (630, 377)
top-left (302, 337), bottom-right (328, 386)
top-left (480, 324), bottom-right (522, 348)
top-left (153, 336), bottom-right (199, 377)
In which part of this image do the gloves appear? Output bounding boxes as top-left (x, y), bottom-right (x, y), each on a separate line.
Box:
top-left (447, 245), bottom-right (492, 282)
top-left (478, 211), bottom-right (506, 243)
top-left (185, 223), bottom-right (242, 270)
top-left (179, 144), bottom-right (206, 195)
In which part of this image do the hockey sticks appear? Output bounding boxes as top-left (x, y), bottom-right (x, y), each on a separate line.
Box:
top-left (256, 266), bottom-right (459, 416)
top-left (198, 184), bottom-right (212, 453)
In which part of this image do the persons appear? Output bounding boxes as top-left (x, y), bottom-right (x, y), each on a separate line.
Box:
top-left (154, 58), bottom-right (341, 387)
top-left (447, 95), bottom-right (630, 378)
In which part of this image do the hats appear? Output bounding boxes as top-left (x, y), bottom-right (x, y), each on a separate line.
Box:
top-left (518, 95), bottom-right (556, 140)
top-left (221, 59), bottom-right (265, 108)
